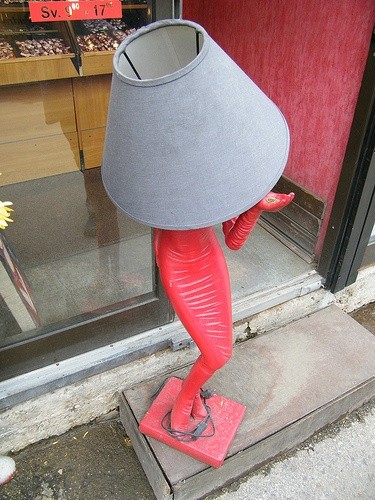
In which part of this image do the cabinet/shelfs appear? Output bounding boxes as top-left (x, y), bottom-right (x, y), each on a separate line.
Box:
top-left (0, 0), bottom-right (155, 189)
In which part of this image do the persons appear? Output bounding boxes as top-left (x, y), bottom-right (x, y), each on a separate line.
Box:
top-left (139, 192), bottom-right (295, 439)
